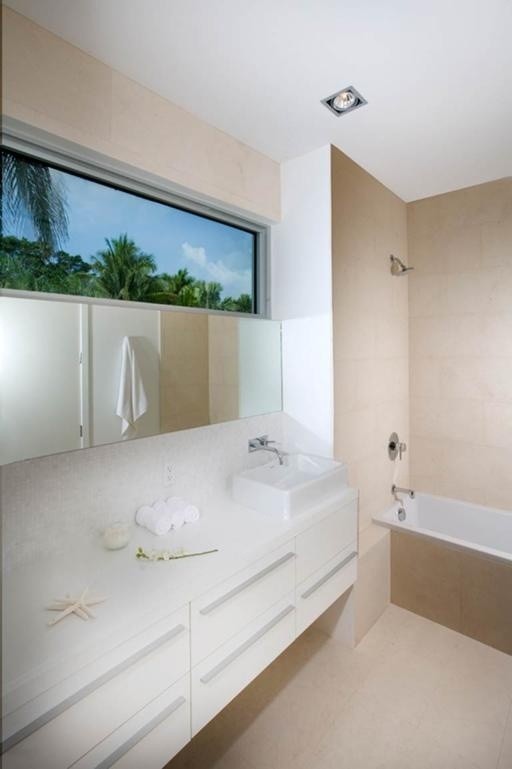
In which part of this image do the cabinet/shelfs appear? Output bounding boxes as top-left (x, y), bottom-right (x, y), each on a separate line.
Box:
top-left (1, 493), bottom-right (358, 768)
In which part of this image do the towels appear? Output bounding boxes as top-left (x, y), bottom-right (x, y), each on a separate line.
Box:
top-left (114, 335), bottom-right (149, 435)
top-left (132, 495), bottom-right (200, 536)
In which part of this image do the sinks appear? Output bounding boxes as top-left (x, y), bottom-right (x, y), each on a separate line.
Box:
top-left (234, 452), bottom-right (348, 516)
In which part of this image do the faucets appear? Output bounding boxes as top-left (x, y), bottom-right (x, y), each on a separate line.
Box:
top-left (393, 484), bottom-right (415, 500)
top-left (247, 433), bottom-right (284, 468)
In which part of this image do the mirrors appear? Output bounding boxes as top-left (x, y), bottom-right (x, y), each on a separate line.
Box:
top-left (0, 298), bottom-right (282, 471)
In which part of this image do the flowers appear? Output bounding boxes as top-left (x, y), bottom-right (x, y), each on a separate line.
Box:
top-left (136, 544), bottom-right (226, 562)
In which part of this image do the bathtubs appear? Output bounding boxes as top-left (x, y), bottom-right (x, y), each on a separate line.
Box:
top-left (373, 491), bottom-right (512, 657)
